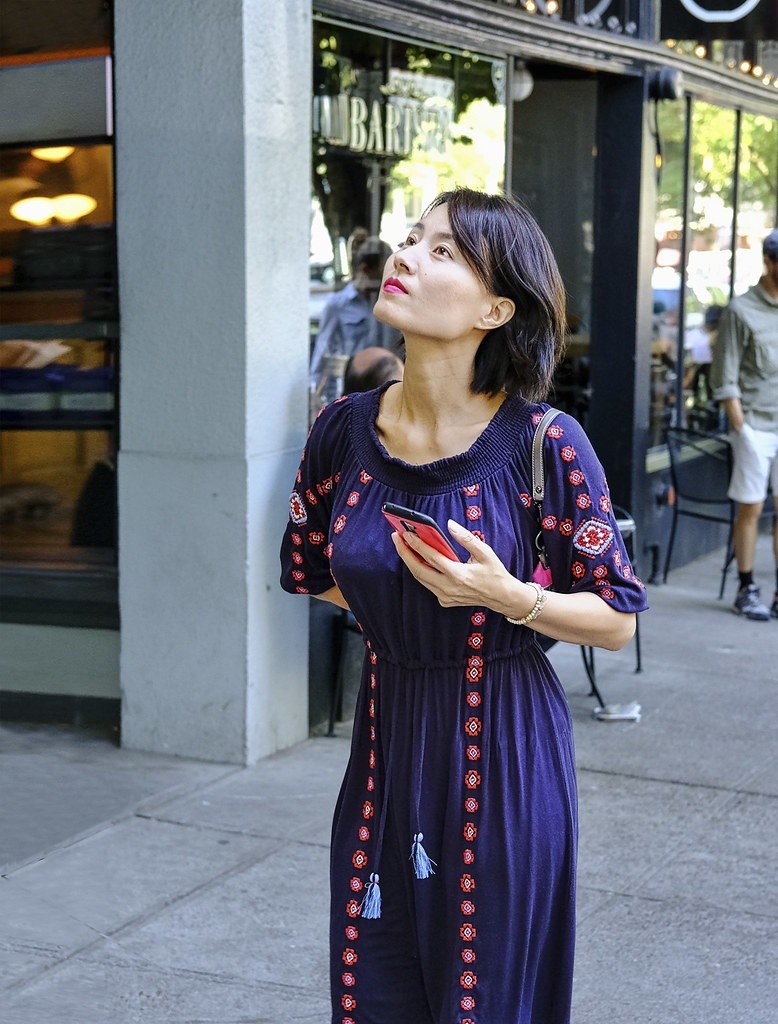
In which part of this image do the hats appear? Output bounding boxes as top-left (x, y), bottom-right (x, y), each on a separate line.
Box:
top-left (763, 231), bottom-right (778, 253)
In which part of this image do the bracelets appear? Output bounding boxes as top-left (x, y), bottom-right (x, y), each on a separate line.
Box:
top-left (505, 581), bottom-right (545, 625)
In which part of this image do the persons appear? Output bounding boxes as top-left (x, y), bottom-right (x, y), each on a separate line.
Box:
top-left (712, 234), bottom-right (778, 622)
top-left (312, 228), bottom-right (403, 393)
top-left (651, 300), bottom-right (721, 404)
top-left (279, 189), bottom-right (647, 1023)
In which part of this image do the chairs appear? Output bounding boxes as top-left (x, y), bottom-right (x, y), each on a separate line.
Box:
top-left (579, 503), bottom-right (645, 716)
top-left (665, 426), bottom-right (747, 599)
top-left (324, 601), bottom-right (360, 741)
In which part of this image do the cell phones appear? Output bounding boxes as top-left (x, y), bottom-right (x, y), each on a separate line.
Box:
top-left (381, 502), bottom-right (465, 573)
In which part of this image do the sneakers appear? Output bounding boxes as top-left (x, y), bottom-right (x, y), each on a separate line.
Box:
top-left (729, 582), bottom-right (778, 621)
